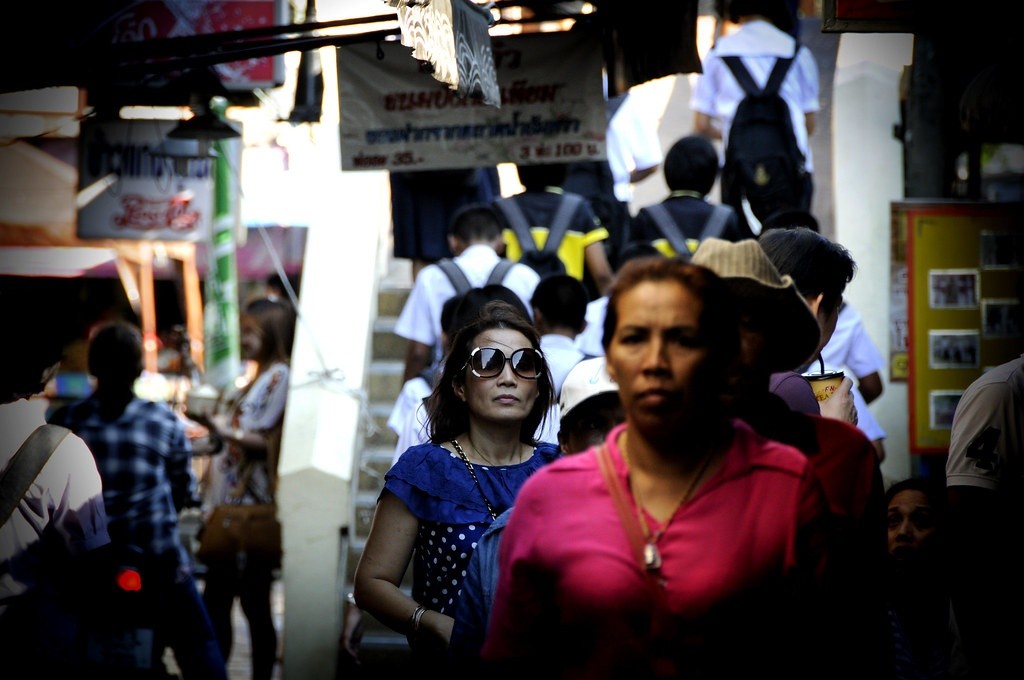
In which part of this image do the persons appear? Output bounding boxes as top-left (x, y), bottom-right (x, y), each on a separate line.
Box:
top-left (0, 135), bottom-right (1024, 680)
top-left (698, 0), bottom-right (823, 229)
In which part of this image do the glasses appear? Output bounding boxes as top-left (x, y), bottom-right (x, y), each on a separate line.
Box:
top-left (459, 347), bottom-right (545, 379)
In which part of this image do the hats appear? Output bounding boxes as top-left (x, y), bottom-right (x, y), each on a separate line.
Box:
top-left (560, 357), bottom-right (620, 426)
top-left (688, 237), bottom-right (821, 374)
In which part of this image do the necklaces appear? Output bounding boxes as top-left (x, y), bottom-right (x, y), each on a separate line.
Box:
top-left (622, 440), bottom-right (712, 568)
top-left (468, 434), bottom-right (522, 467)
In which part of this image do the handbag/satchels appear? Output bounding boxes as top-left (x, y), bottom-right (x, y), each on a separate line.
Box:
top-left (193, 429), bottom-right (284, 573)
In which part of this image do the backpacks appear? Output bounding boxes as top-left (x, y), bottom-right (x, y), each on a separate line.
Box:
top-left (721, 40), bottom-right (805, 211)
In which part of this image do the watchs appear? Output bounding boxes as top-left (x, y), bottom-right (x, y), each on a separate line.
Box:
top-left (406, 607), bottom-right (426, 652)
